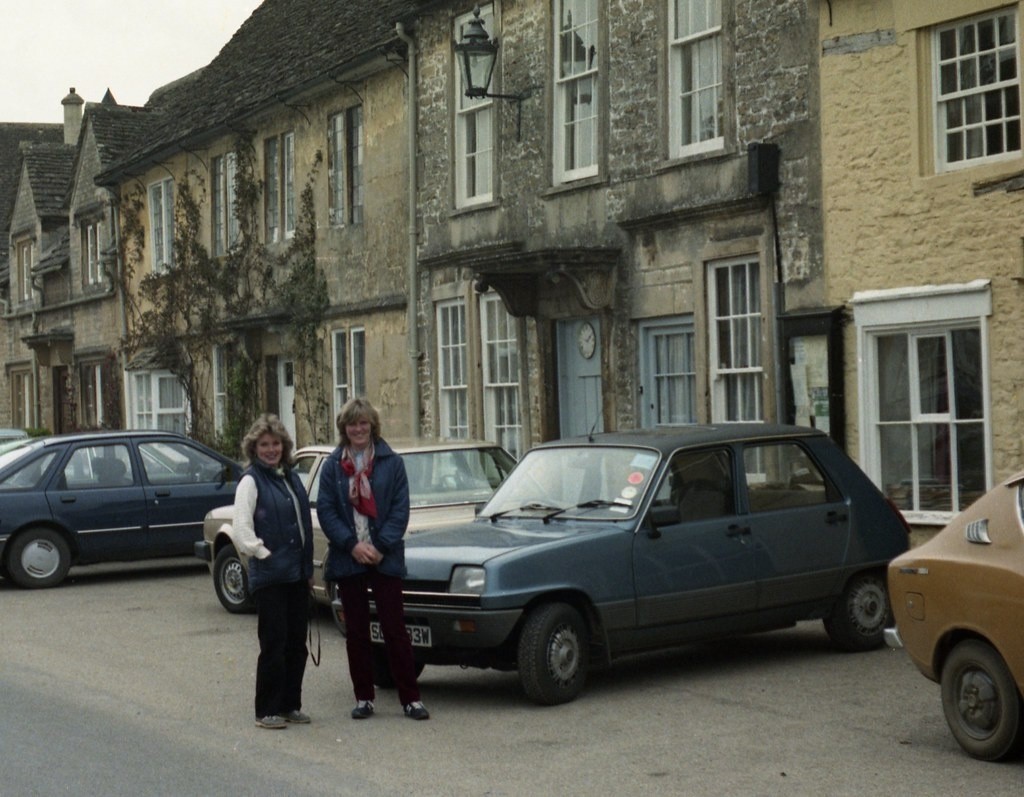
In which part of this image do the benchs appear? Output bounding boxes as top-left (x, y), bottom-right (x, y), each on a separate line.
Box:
top-left (679, 489), bottom-right (822, 520)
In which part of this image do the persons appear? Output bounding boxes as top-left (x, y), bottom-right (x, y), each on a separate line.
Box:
top-left (232, 415), bottom-right (313, 729)
top-left (316, 399), bottom-right (430, 720)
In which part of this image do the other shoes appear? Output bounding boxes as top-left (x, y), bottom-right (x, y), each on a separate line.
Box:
top-left (255, 714), bottom-right (287, 728)
top-left (352, 699), bottom-right (375, 718)
top-left (404, 700), bottom-right (429, 719)
top-left (279, 710), bottom-right (310, 723)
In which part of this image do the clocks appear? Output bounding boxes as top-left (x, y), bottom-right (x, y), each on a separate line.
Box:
top-left (577, 322), bottom-right (596, 359)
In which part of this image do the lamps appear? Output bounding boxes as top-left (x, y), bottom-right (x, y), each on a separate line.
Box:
top-left (450, 2), bottom-right (531, 144)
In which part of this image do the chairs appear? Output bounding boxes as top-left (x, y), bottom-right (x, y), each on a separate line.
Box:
top-left (90, 458), bottom-right (132, 486)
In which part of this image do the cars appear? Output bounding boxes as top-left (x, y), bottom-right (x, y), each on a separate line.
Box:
top-left (0, 426), bottom-right (209, 489)
top-left (330, 423), bottom-right (913, 707)
top-left (887, 470), bottom-right (1023, 763)
top-left (193, 437), bottom-right (563, 620)
top-left (0, 428), bottom-right (244, 591)
